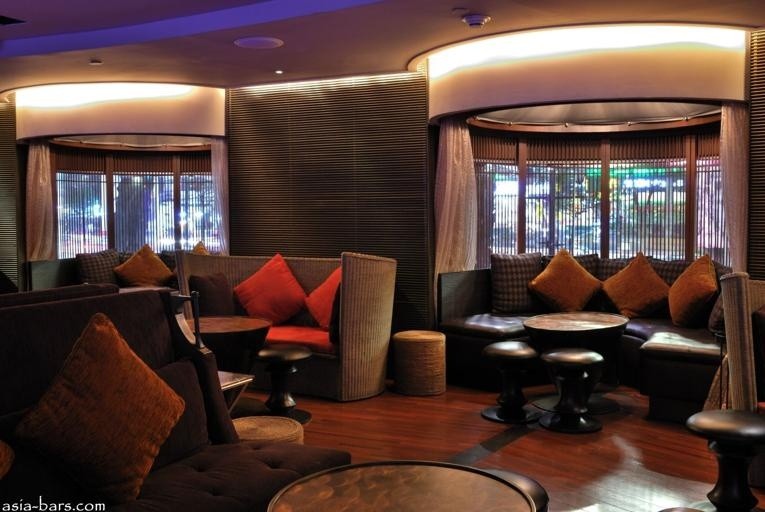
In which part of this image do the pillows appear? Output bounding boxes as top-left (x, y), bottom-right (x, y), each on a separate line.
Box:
top-left (189, 255), bottom-right (342, 347)
top-left (486, 250), bottom-right (728, 334)
top-left (74, 241), bottom-right (212, 286)
top-left (10, 308), bottom-right (186, 508)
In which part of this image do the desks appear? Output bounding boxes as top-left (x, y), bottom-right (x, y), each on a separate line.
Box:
top-left (189, 315), bottom-right (285, 376)
top-left (267, 452), bottom-right (552, 512)
top-left (522, 310), bottom-right (637, 419)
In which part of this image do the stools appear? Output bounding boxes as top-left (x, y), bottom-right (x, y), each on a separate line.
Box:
top-left (255, 345), bottom-right (315, 425)
top-left (478, 339), bottom-right (607, 436)
top-left (686, 408), bottom-right (764, 512)
top-left (391, 326), bottom-right (448, 399)
top-left (232, 414), bottom-right (304, 450)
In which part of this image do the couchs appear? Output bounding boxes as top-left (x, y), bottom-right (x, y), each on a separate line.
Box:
top-left (180, 248), bottom-right (401, 403)
top-left (437, 259), bottom-right (736, 423)
top-left (23, 246), bottom-right (222, 290)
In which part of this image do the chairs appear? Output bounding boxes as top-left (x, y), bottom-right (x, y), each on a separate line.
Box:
top-left (2, 288), bottom-right (349, 512)
